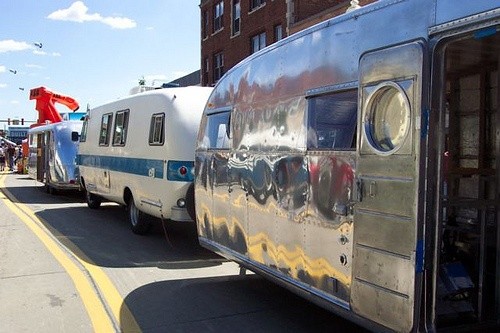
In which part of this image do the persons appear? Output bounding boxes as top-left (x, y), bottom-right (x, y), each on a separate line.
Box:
top-left (0, 146), bottom-right (5, 171)
top-left (7, 143), bottom-right (15, 171)
top-left (18, 145), bottom-right (23, 173)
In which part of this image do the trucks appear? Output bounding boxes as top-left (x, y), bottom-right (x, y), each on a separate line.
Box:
top-left (193, 0), bottom-right (500, 332)
top-left (72, 75), bottom-right (215, 238)
top-left (26, 111), bottom-right (87, 194)
